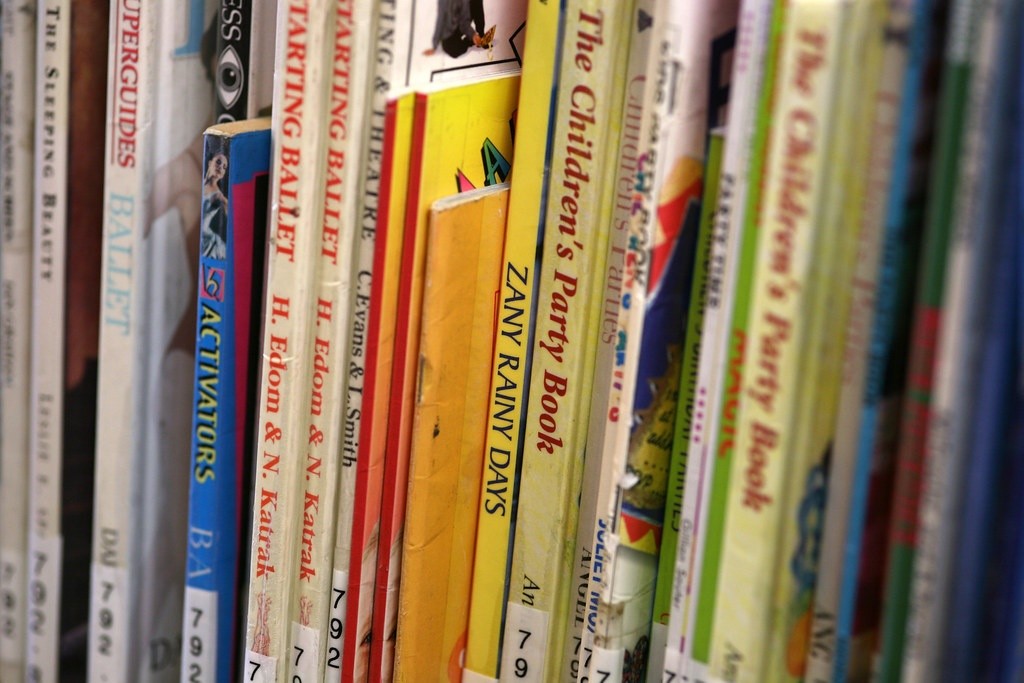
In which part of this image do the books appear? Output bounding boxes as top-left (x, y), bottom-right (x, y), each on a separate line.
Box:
top-left (0, 0), bottom-right (1024, 683)
